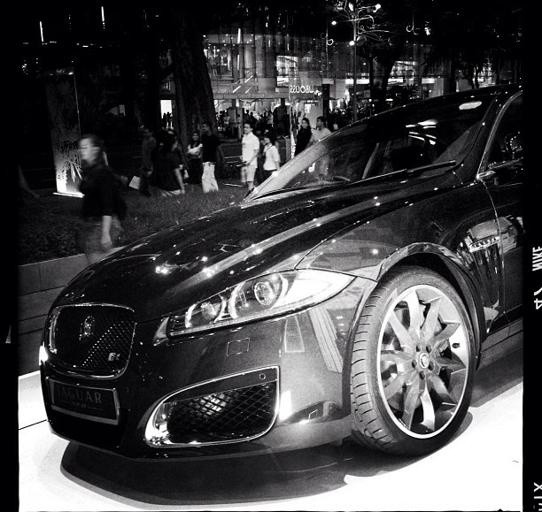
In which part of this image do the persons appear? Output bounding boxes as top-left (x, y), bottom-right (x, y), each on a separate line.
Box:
top-left (132, 98), bottom-right (375, 197)
top-left (78, 135), bottom-right (126, 263)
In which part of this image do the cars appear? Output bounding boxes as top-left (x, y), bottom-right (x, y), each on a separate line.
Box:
top-left (37, 83), bottom-right (525, 465)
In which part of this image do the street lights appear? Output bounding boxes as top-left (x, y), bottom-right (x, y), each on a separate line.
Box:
top-left (337, 1), bottom-right (381, 123)
top-left (47, 71), bottom-right (82, 134)
top-left (326, 21), bottom-right (337, 78)
